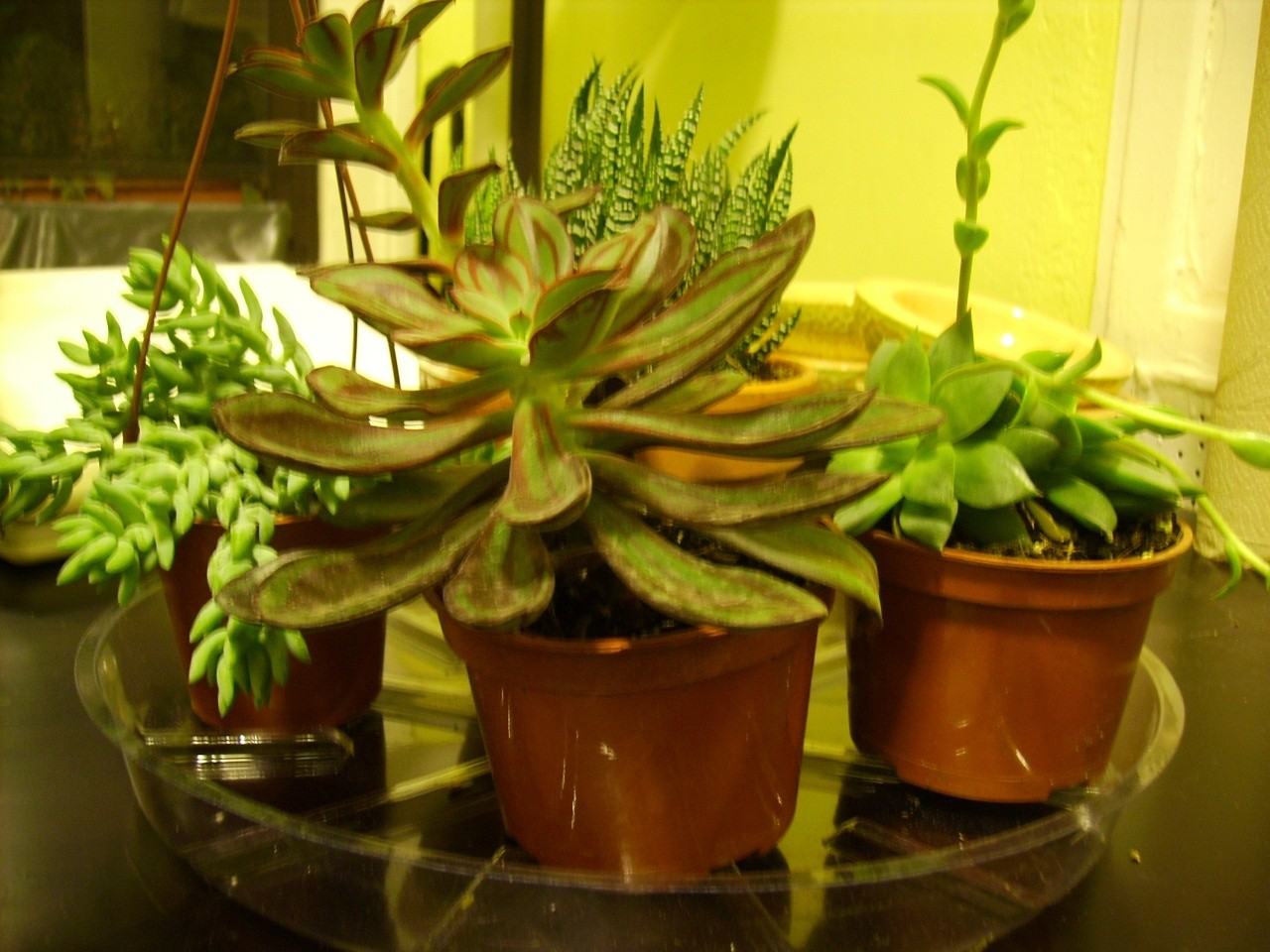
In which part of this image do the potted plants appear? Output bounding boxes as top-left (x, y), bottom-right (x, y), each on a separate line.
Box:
top-left (0, 0), bottom-right (1270, 882)
